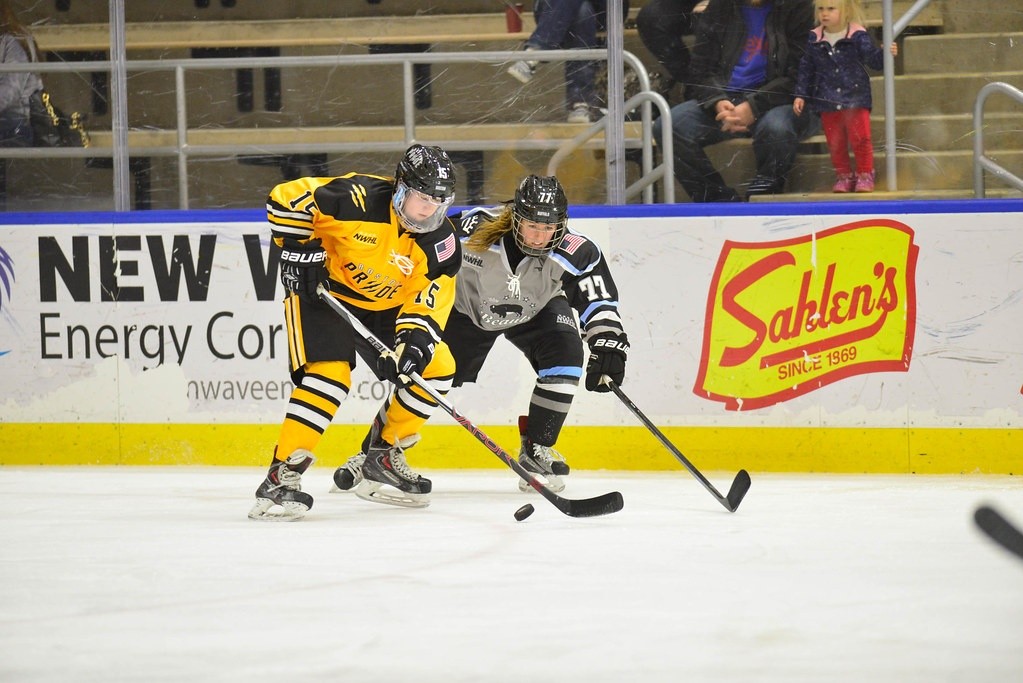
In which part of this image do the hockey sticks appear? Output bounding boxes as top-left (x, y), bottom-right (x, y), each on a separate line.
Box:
top-left (316, 281), bottom-right (625, 519)
top-left (972, 506), bottom-right (1023, 559)
top-left (602, 373), bottom-right (751, 513)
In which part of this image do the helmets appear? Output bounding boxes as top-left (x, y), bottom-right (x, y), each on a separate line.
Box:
top-left (392, 144), bottom-right (456, 196)
top-left (512, 174), bottom-right (570, 259)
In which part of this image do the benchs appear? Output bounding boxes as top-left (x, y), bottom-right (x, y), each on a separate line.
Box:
top-left (16, 0), bottom-right (947, 213)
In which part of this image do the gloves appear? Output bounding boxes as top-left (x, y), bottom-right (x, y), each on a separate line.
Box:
top-left (377, 328), bottom-right (436, 389)
top-left (585, 337), bottom-right (631, 393)
top-left (279, 237), bottom-right (330, 305)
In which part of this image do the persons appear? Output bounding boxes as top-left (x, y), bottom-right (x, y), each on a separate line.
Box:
top-left (331, 175), bottom-right (632, 493)
top-left (0, 35), bottom-right (89, 170)
top-left (508, 0), bottom-right (631, 122)
top-left (248, 144), bottom-right (463, 521)
top-left (637, 0), bottom-right (901, 203)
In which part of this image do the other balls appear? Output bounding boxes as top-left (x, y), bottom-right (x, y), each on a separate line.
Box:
top-left (514, 504), bottom-right (534, 522)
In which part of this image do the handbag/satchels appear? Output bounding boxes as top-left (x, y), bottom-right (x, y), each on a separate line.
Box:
top-left (28, 87), bottom-right (91, 148)
top-left (592, 66), bottom-right (670, 123)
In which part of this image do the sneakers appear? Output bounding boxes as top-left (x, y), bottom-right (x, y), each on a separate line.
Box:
top-left (567, 103), bottom-right (589, 122)
top-left (247, 445), bottom-right (313, 523)
top-left (356, 425), bottom-right (431, 508)
top-left (832, 169), bottom-right (874, 194)
top-left (711, 189), bottom-right (739, 202)
top-left (747, 175), bottom-right (786, 195)
top-left (328, 450), bottom-right (366, 491)
top-left (507, 60), bottom-right (536, 84)
top-left (518, 442), bottom-right (570, 493)
